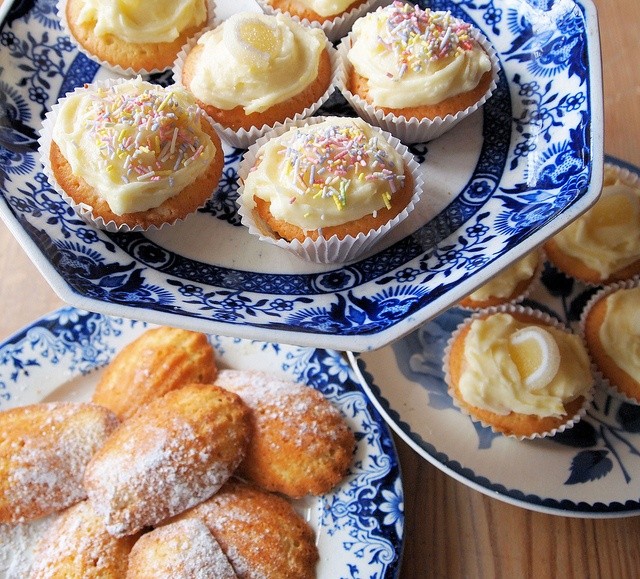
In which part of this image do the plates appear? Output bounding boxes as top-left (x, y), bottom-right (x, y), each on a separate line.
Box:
top-left (0, 0), bottom-right (604, 353)
top-left (346, 152), bottom-right (640, 520)
top-left (0, 304), bottom-right (406, 579)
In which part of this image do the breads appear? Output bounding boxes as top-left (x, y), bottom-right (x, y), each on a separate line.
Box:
top-left (128, 518), bottom-right (236, 579)
top-left (217, 367), bottom-right (353, 499)
top-left (1, 402), bottom-right (123, 533)
top-left (155, 482), bottom-right (317, 579)
top-left (94, 325), bottom-right (217, 425)
top-left (32, 499), bottom-right (144, 579)
top-left (84, 383), bottom-right (251, 537)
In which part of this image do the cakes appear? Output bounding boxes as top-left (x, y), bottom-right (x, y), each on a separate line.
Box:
top-left (443, 305), bottom-right (595, 440)
top-left (56, 0), bottom-right (217, 77)
top-left (38, 77), bottom-right (225, 233)
top-left (254, 0), bottom-right (375, 42)
top-left (453, 245), bottom-right (546, 310)
top-left (578, 277), bottom-right (640, 408)
top-left (173, 13), bottom-right (337, 149)
top-left (543, 163), bottom-right (639, 287)
top-left (237, 116), bottom-right (424, 266)
top-left (337, 1), bottom-right (500, 146)
